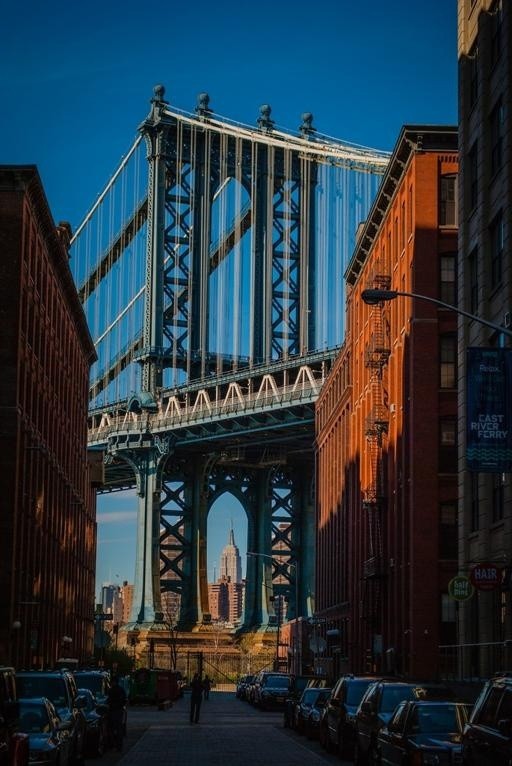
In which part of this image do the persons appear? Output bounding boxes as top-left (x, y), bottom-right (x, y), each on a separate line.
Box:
top-left (190, 672), bottom-right (214, 724)
top-left (108, 677), bottom-right (125, 753)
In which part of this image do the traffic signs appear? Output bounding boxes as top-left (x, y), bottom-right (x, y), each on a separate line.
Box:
top-left (306, 617), bottom-right (326, 625)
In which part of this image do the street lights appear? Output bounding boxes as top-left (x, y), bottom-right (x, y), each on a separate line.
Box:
top-left (360, 289), bottom-right (512, 337)
top-left (241, 552), bottom-right (299, 676)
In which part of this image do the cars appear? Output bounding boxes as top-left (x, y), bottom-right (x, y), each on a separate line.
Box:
top-left (0, 666), bottom-right (126, 766)
top-left (236, 672), bottom-right (512, 766)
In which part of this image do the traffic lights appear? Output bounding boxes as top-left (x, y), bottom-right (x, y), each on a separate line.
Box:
top-left (112, 624), bottom-right (117, 633)
top-left (173, 625), bottom-right (181, 631)
top-left (154, 621), bottom-right (165, 624)
